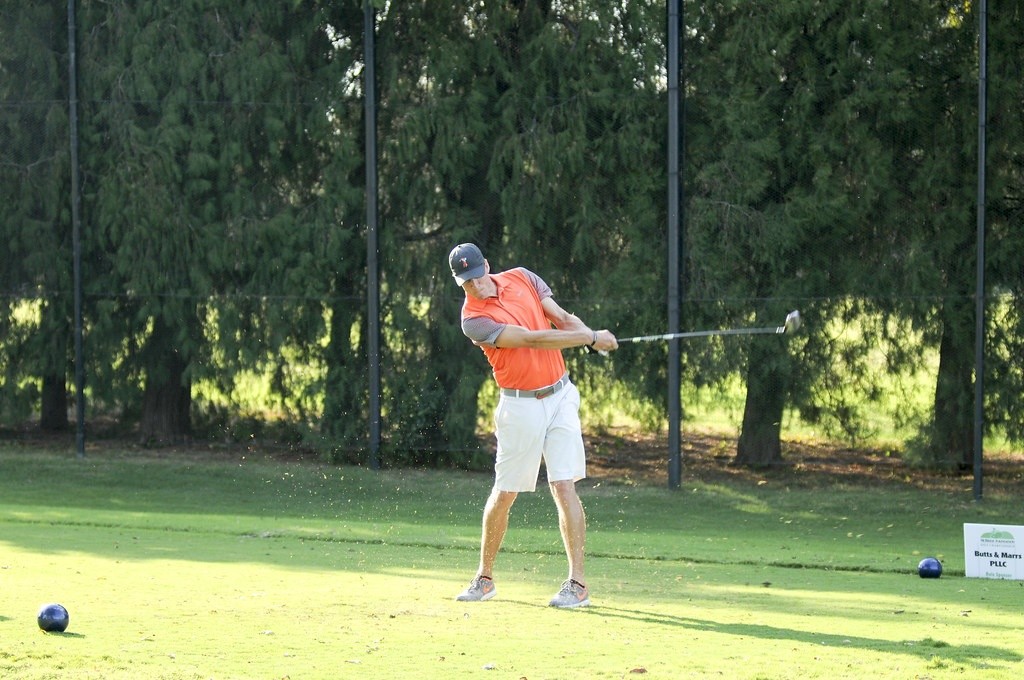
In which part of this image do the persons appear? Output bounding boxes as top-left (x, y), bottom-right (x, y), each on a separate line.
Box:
top-left (449, 244), bottom-right (619, 609)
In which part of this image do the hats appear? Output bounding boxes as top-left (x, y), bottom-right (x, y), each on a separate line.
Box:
top-left (448, 243), bottom-right (485, 287)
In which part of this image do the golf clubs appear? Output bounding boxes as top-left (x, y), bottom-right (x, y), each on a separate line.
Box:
top-left (584, 309), bottom-right (802, 355)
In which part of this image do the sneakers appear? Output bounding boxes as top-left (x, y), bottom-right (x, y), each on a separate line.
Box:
top-left (456, 576), bottom-right (497, 600)
top-left (550, 579), bottom-right (590, 608)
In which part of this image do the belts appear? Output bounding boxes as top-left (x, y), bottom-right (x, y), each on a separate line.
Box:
top-left (503, 375), bottom-right (568, 399)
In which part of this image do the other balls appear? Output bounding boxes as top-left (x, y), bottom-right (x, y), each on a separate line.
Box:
top-left (37, 604), bottom-right (70, 633)
top-left (918, 557), bottom-right (943, 578)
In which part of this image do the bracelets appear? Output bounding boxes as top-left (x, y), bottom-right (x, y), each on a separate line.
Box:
top-left (591, 332), bottom-right (597, 346)
